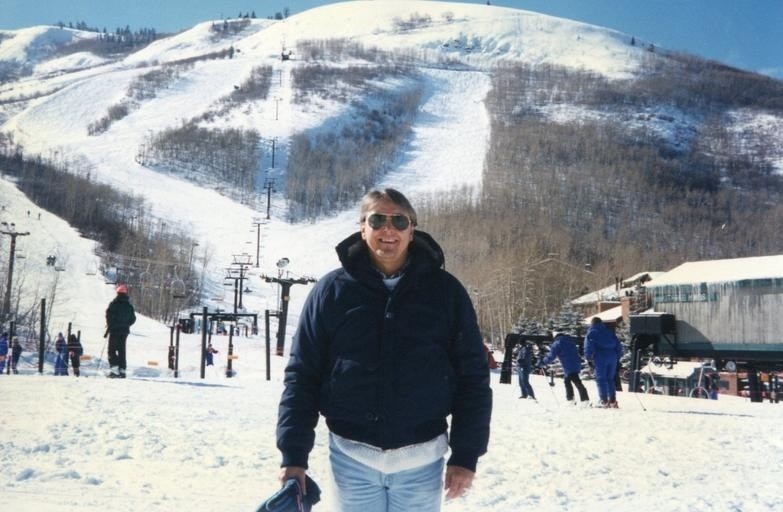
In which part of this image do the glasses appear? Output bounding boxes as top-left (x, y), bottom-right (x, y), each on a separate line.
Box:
top-left (367, 213), bottom-right (412, 232)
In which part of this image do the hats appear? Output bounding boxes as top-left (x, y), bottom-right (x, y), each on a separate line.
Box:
top-left (116, 285), bottom-right (128, 293)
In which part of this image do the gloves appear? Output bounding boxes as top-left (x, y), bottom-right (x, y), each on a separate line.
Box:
top-left (254, 472), bottom-right (322, 512)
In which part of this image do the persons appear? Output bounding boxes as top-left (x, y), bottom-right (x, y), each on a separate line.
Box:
top-left (11, 336), bottom-right (22, 374)
top-left (517, 337), bottom-right (536, 399)
top-left (69, 334), bottom-right (82, 376)
top-left (0, 332), bottom-right (9, 373)
top-left (584, 317), bottom-right (619, 407)
top-left (276, 186), bottom-right (492, 512)
top-left (105, 284), bottom-right (136, 379)
top-left (52, 333), bottom-right (67, 376)
top-left (542, 333), bottom-right (589, 403)
top-left (206, 343), bottom-right (218, 365)
